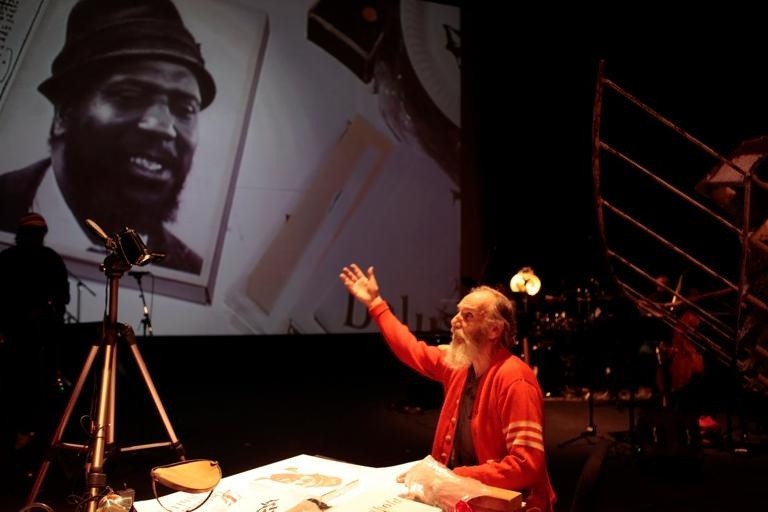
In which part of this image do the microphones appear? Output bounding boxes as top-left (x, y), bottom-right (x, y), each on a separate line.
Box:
top-left (128, 271), bottom-right (149, 277)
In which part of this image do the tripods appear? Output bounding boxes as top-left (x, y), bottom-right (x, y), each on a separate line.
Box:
top-left (22, 219), bottom-right (186, 511)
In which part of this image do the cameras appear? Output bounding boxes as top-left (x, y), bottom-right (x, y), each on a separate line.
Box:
top-left (102, 226), bottom-right (167, 280)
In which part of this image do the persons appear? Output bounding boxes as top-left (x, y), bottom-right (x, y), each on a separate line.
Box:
top-left (340, 262), bottom-right (555, 512)
top-left (0, 213), bottom-right (70, 444)
top-left (651, 267), bottom-right (714, 479)
top-left (0, 1), bottom-right (217, 275)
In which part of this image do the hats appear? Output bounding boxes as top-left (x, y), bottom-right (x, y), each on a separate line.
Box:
top-left (36, 0), bottom-right (217, 111)
